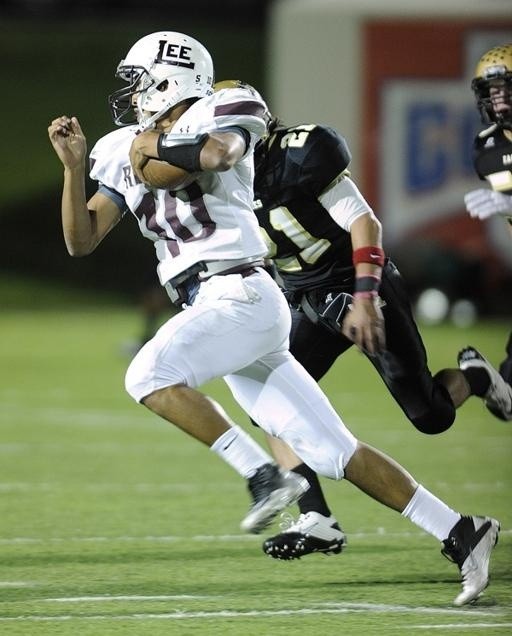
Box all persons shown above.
[465,45,512,386]
[212,80,512,561]
[47,30,501,607]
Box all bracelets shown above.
[354,276,380,297]
[352,246,384,267]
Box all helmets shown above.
[471,43,512,89]
[115,31,266,131]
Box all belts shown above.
[296,292,319,324]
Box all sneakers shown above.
[457,346,512,422]
[262,510,348,561]
[239,462,311,534]
[440,512,501,606]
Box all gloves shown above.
[342,298,387,358]
[463,188,512,221]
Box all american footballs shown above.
[142,157,203,191]
